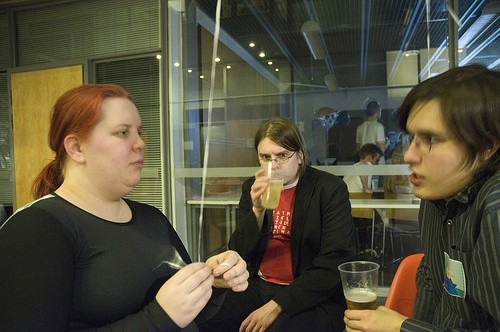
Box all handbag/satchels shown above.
[383,143,414,193]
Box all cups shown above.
[338,260,380,312]
[256,161,284,209]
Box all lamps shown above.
[301,19,327,61]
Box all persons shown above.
[343,63,500,332]
[0,83,215,332]
[329,109,357,163]
[342,143,384,226]
[356,101,387,166]
[199,115,360,332]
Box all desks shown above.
[186,196,421,249]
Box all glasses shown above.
[256,151,296,165]
[402,129,464,155]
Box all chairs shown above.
[348,192,424,287]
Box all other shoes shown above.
[358,249,377,260]
[375,247,384,258]
